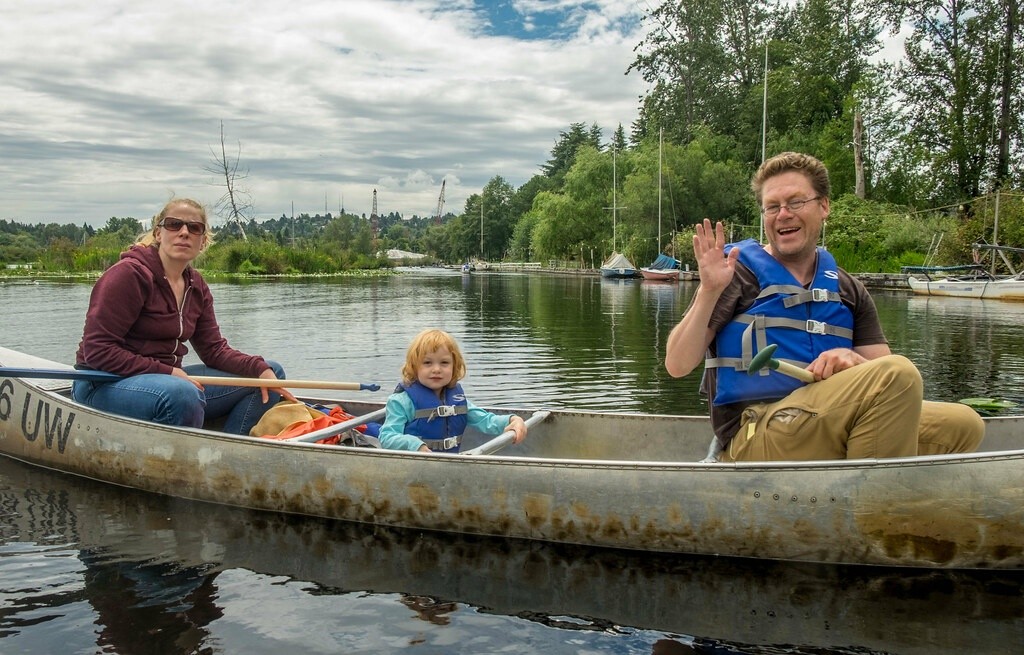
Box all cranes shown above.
[437,178,447,218]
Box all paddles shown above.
[747,342,822,384]
[0,367,382,393]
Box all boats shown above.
[908,190,1024,300]
[1,343,1024,574]
[0,448,1023,655]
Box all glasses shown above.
[761,197,822,218]
[156,217,205,235]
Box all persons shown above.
[379,329,527,452]
[665,152,986,463]
[70,198,298,436]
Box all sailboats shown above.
[640,127,696,281]
[599,131,640,279]
[472,203,493,273]
